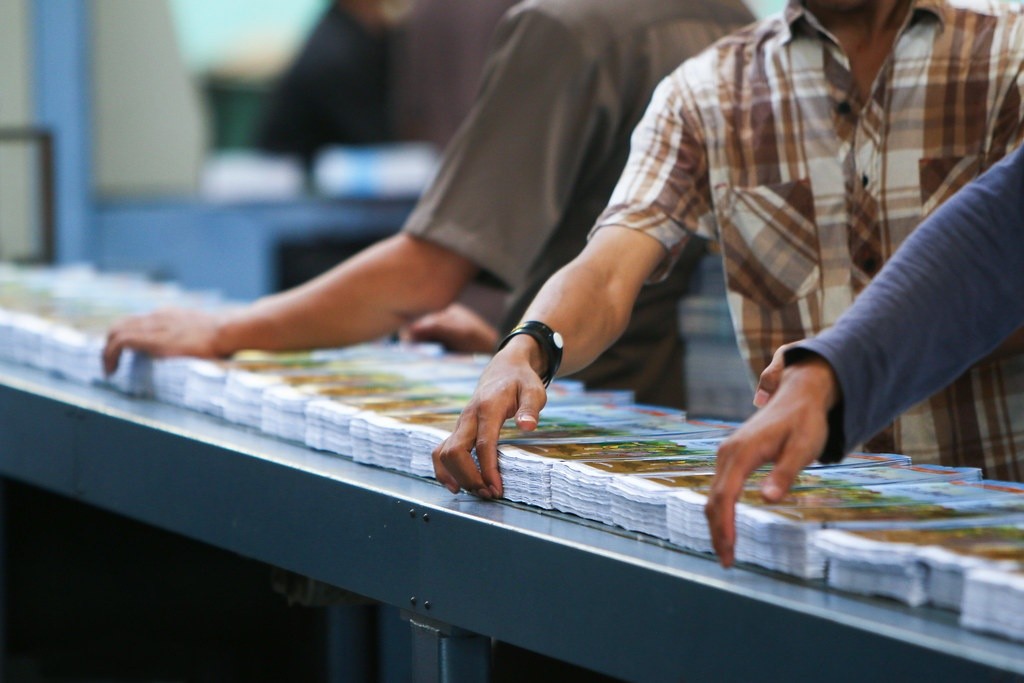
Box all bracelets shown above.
[491,321,563,390]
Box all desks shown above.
[85,193,421,311]
[0,356,1024,683]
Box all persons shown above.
[432,0,1024,499]
[103,1,757,411]
[705,140,1024,570]
